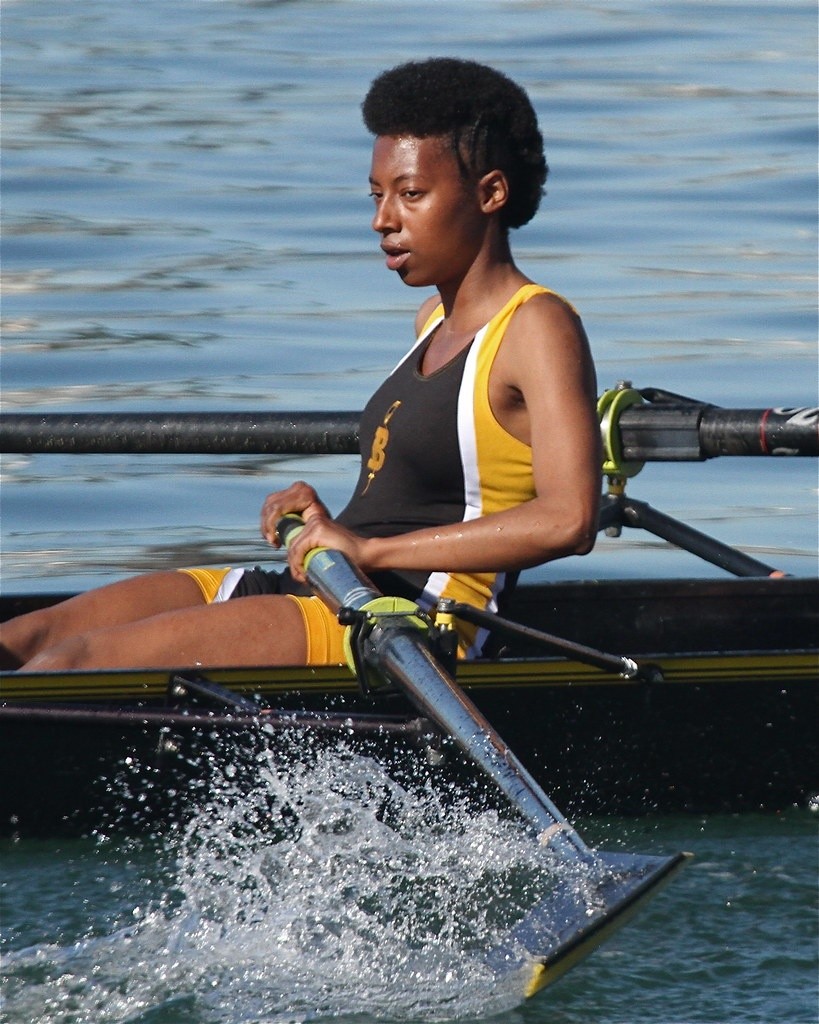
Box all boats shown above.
[1,408,819,830]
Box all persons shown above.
[0,57,600,672]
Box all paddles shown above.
[271,512,694,1004]
[0,403,819,462]
